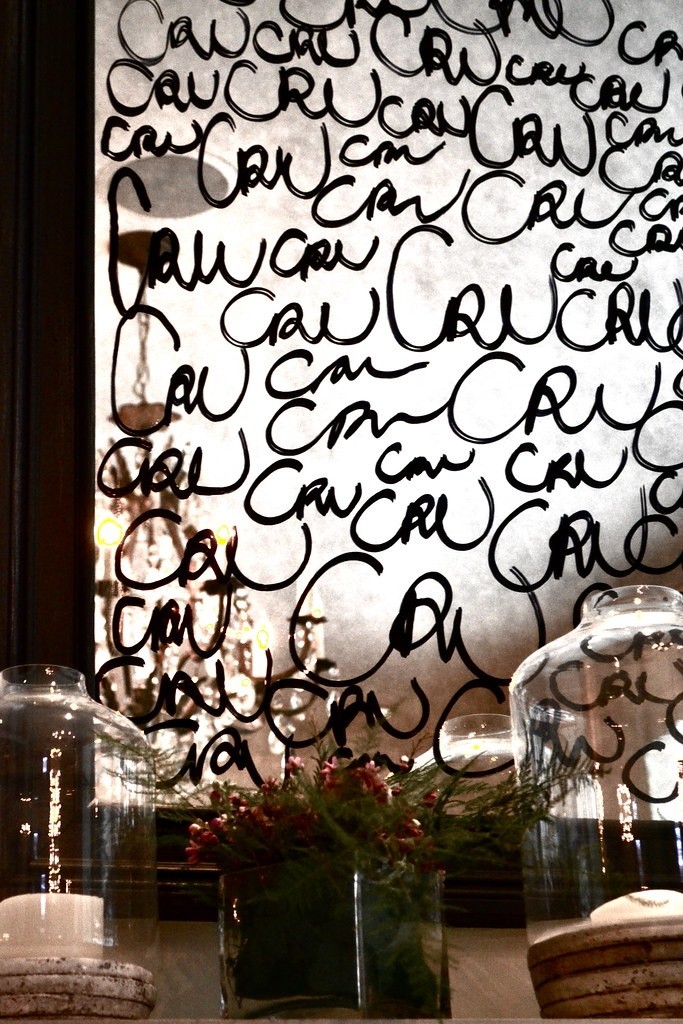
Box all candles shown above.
[589,890,683,923]
[0,893,104,961]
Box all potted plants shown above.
[84,692,614,1024]
